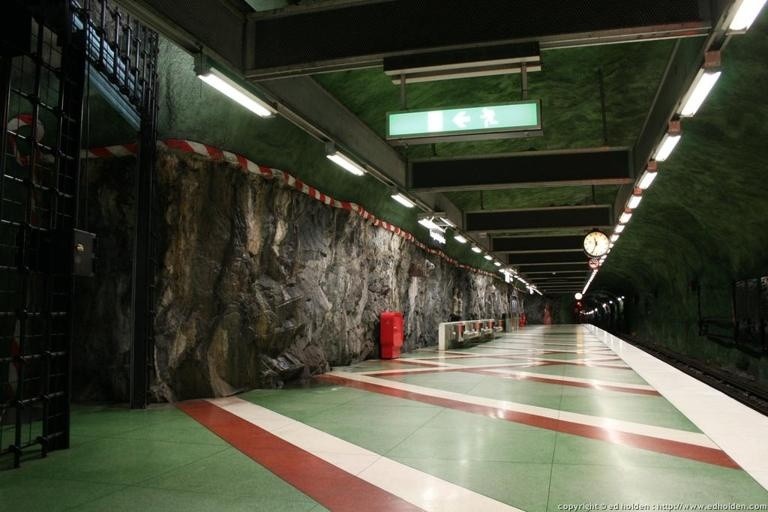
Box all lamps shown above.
[389,187,417,209]
[626,193,643,210]
[192,55,279,121]
[324,139,369,177]
[675,64,723,118]
[417,217,446,236]
[636,169,658,190]
[651,130,682,163]
[451,229,544,296]
[581,209,633,295]
[723,0,768,36]
